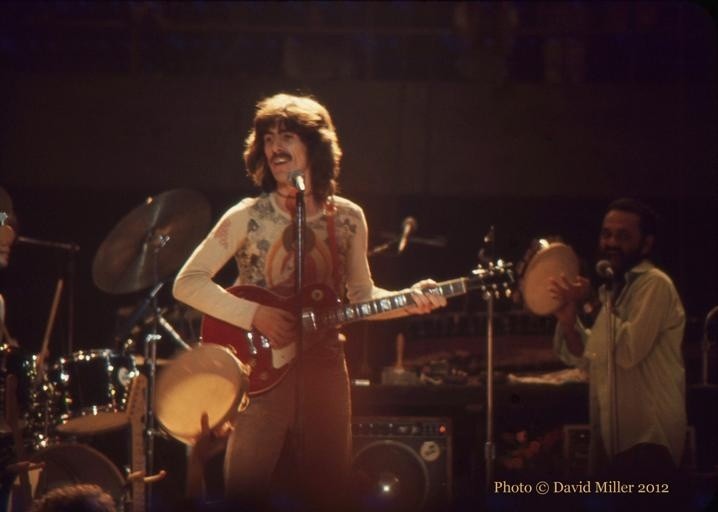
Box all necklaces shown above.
[274,188,314,199]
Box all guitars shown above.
[201,256,517,396]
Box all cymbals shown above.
[91,189,211,294]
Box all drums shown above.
[518,239,580,316]
[6,441,132,512]
[149,341,251,446]
[49,348,138,436]
[0,344,42,434]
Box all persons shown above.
[548,197,687,512]
[0,196,16,334]
[172,93,446,507]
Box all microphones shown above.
[595,259,615,280]
[286,168,308,194]
[396,215,417,254]
[478,224,496,263]
